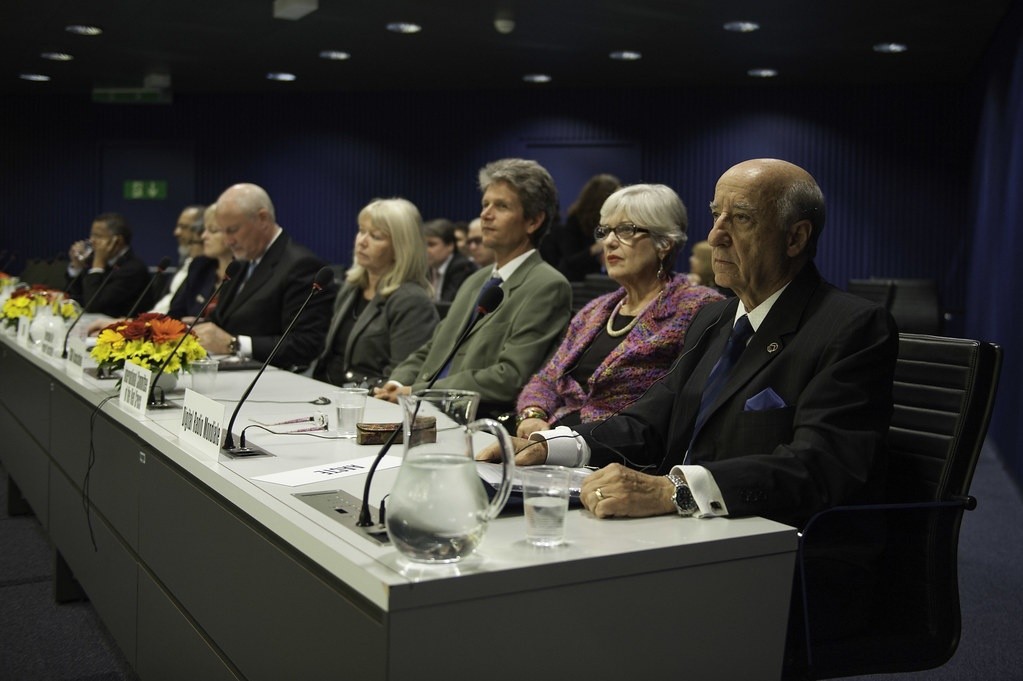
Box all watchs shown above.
[229,336,240,356]
[666,474,699,516]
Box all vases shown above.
[122,362,179,397]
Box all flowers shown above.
[1,283,76,328]
[91,312,211,379]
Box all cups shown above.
[188,359,219,394]
[334,387,369,435]
[523,464,572,546]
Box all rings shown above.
[596,489,604,499]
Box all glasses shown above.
[466,237,483,248]
[594,223,651,241]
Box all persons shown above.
[548,173,621,274]
[467,218,495,267]
[180,183,336,371]
[373,159,574,430]
[140,202,247,325]
[473,159,900,656]
[513,183,723,438]
[688,241,737,297]
[65,211,150,318]
[423,219,477,320]
[454,223,468,255]
[302,198,439,397]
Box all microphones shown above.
[126,257,171,322]
[64,253,95,293]
[224,266,336,450]
[358,285,504,527]
[148,261,243,404]
[62,259,126,358]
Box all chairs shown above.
[433,262,1004,681]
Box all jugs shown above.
[385,389,515,564]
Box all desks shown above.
[0,315,800,681]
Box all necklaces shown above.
[353,305,357,320]
[606,295,659,337]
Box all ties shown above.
[679,313,754,465]
[233,262,257,301]
[432,268,441,300]
[434,276,503,380]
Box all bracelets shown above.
[516,408,549,425]
